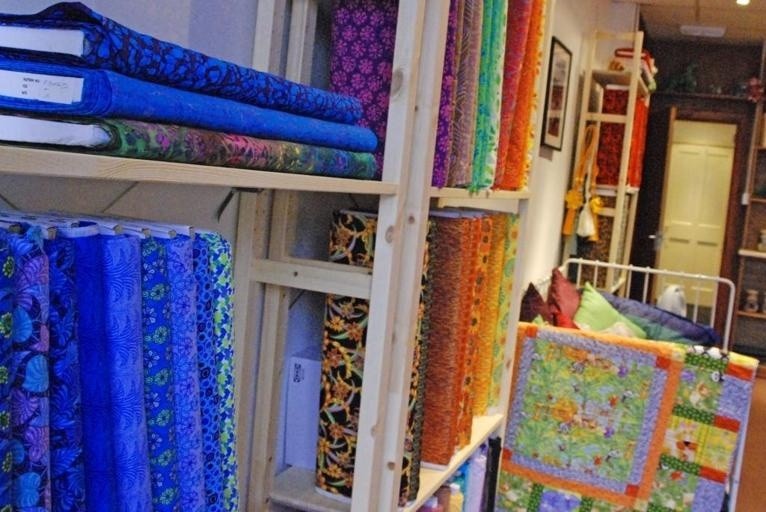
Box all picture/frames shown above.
[541,36,572,151]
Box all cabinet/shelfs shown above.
[573,27,652,292]
[735,149,766,319]
[0,0,553,511]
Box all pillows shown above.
[547,269,582,319]
[572,283,647,339]
[551,305,579,328]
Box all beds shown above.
[494,258,760,512]
